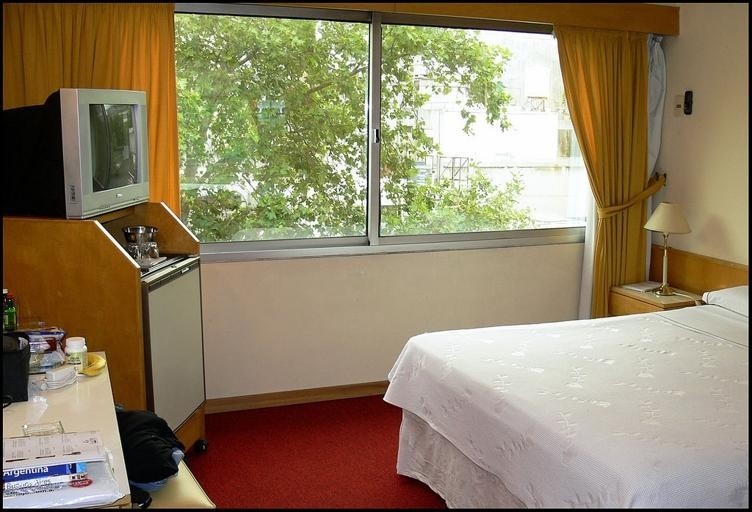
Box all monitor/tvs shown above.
[3,88,149,219]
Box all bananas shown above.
[84,350,106,376]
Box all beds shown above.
[378,273,750,510]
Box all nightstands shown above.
[605,278,702,319]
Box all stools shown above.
[139,449,220,510]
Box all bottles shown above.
[3,287,18,332]
[64,336,89,373]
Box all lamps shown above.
[642,201,692,296]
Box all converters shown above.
[45,365,74,381]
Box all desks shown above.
[0,341,136,510]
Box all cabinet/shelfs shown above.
[2,199,217,461]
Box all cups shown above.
[42,336,57,354]
[127,242,161,269]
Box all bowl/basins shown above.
[122,224,160,243]
